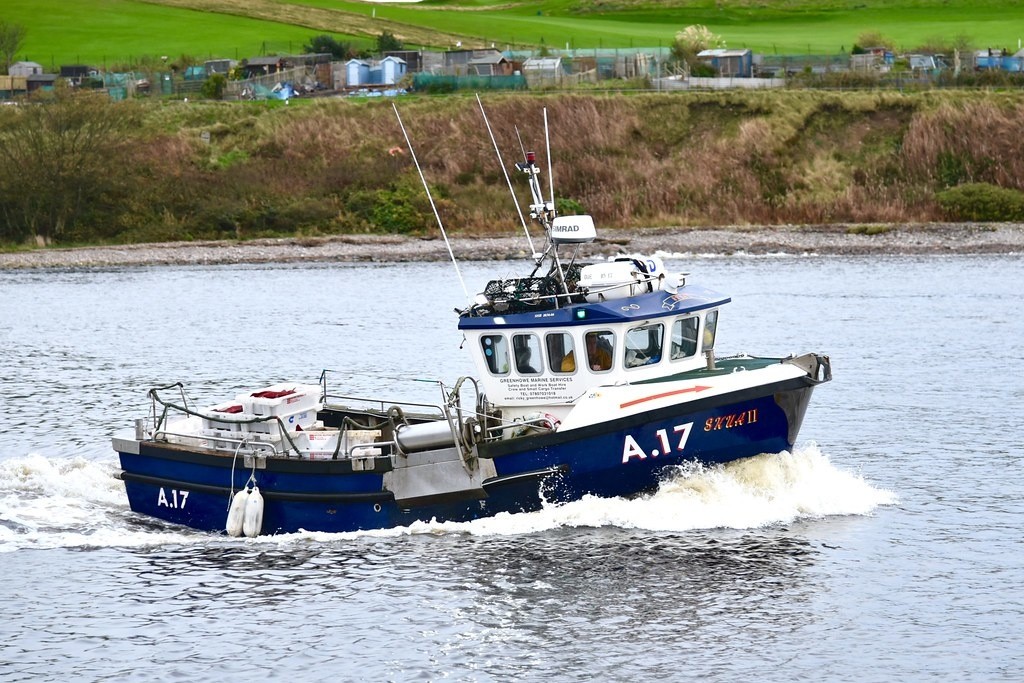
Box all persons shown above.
[500,347,537,373]
[561,335,611,372]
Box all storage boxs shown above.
[202,383,381,458]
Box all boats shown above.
[102,90,835,538]
